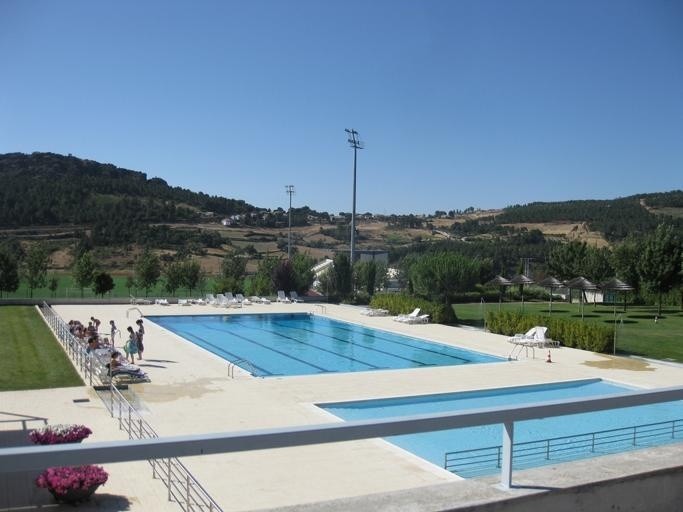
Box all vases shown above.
[46,486,99,504]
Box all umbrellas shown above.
[485,273,634,321]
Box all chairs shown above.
[154,298,169,305]
[177,292,270,308]
[103,364,148,383]
[128,295,152,305]
[276,291,304,303]
[392,308,429,325]
[508,325,560,349]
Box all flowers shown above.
[33,462,109,496]
[25,423,91,445]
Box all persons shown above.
[66,316,144,373]
